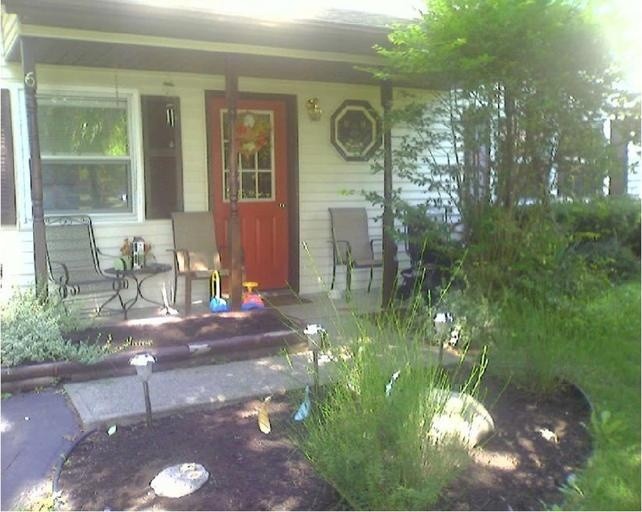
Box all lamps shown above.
[306,96,323,121]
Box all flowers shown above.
[119,236,150,256]
[234,113,266,160]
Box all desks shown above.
[103,262,172,315]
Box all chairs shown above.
[329,207,384,292]
[166,211,245,317]
[43,215,129,321]
